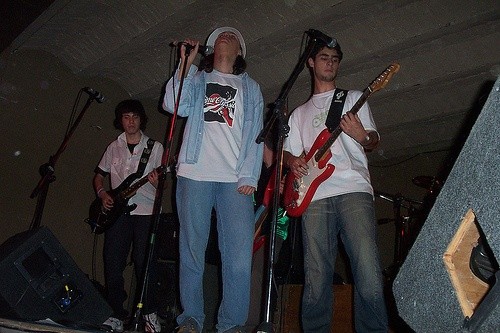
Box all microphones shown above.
[86,87,106,103]
[173,40,214,55]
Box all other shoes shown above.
[222,325,250,333]
[178,316,200,333]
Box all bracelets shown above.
[96,184,106,198]
[360,134,374,146]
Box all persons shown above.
[93,98,171,333]
[283,36,391,333]
[163,27,265,333]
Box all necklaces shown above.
[311,97,332,109]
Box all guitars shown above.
[89,154,178,234]
[252,174,287,251]
[284,63,401,217]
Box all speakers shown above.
[147,259,222,333]
[394,75,500,333]
[0,223,115,328]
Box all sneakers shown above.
[135,313,161,333]
[101,318,124,333]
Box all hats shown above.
[206,26,246,59]
[308,30,343,64]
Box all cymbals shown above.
[411,177,445,192]
